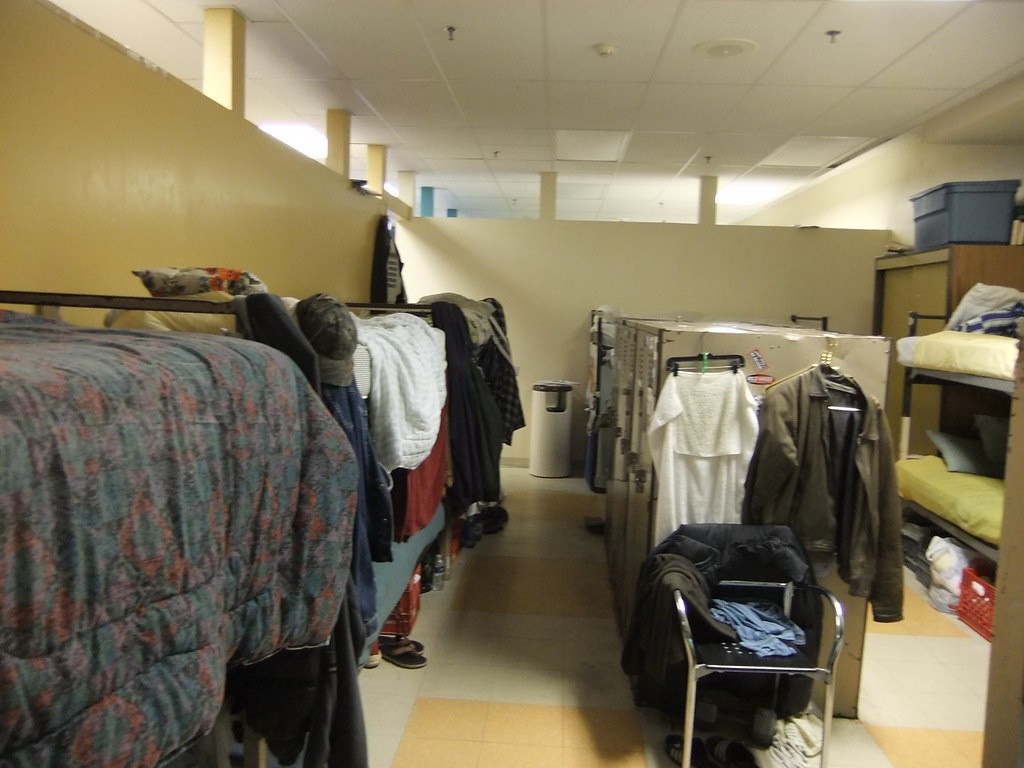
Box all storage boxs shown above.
[381,561,423,637]
[955,569,999,641]
[909,177,1021,248]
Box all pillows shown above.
[129,267,268,295]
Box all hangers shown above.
[667,333,873,413]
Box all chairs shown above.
[669,521,846,767]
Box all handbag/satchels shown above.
[925,535,989,613]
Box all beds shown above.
[899,307,1023,612]
[1,288,528,767]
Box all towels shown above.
[644,370,762,549]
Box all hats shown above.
[296,293,358,386]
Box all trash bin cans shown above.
[529,382,573,477]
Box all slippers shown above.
[706,735,760,768]
[665,734,722,768]
[379,634,424,655]
[381,644,428,668]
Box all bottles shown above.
[431,554,445,590]
